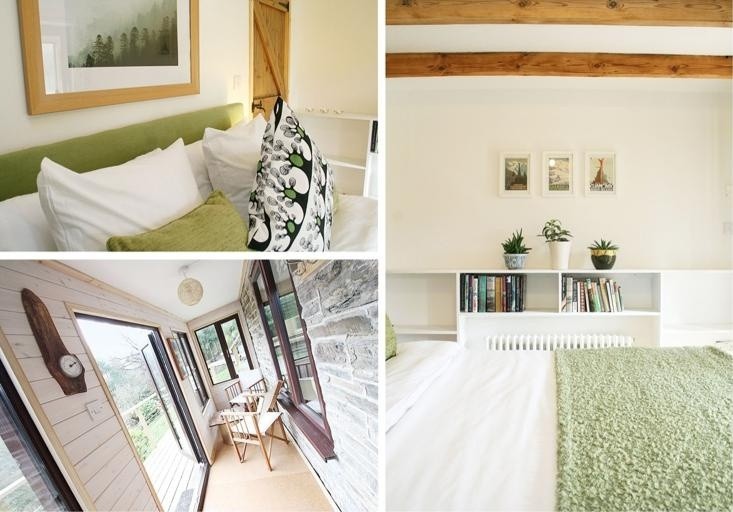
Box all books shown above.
[562,276,625,312]
[460,273,527,312]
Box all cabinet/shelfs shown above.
[295,111,375,197]
[456,270,662,348]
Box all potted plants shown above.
[501,227,533,269]
[536,218,574,270]
[587,237,620,269]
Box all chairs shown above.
[220,378,289,471]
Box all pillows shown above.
[386,315,396,358]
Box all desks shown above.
[209,408,248,445]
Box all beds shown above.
[385,341,732,511]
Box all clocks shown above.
[59,354,82,379]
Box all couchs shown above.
[1,102,376,253]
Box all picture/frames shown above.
[17,1,201,116]
[541,149,575,197]
[584,151,618,197]
[498,150,532,197]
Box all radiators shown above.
[486,335,634,350]
